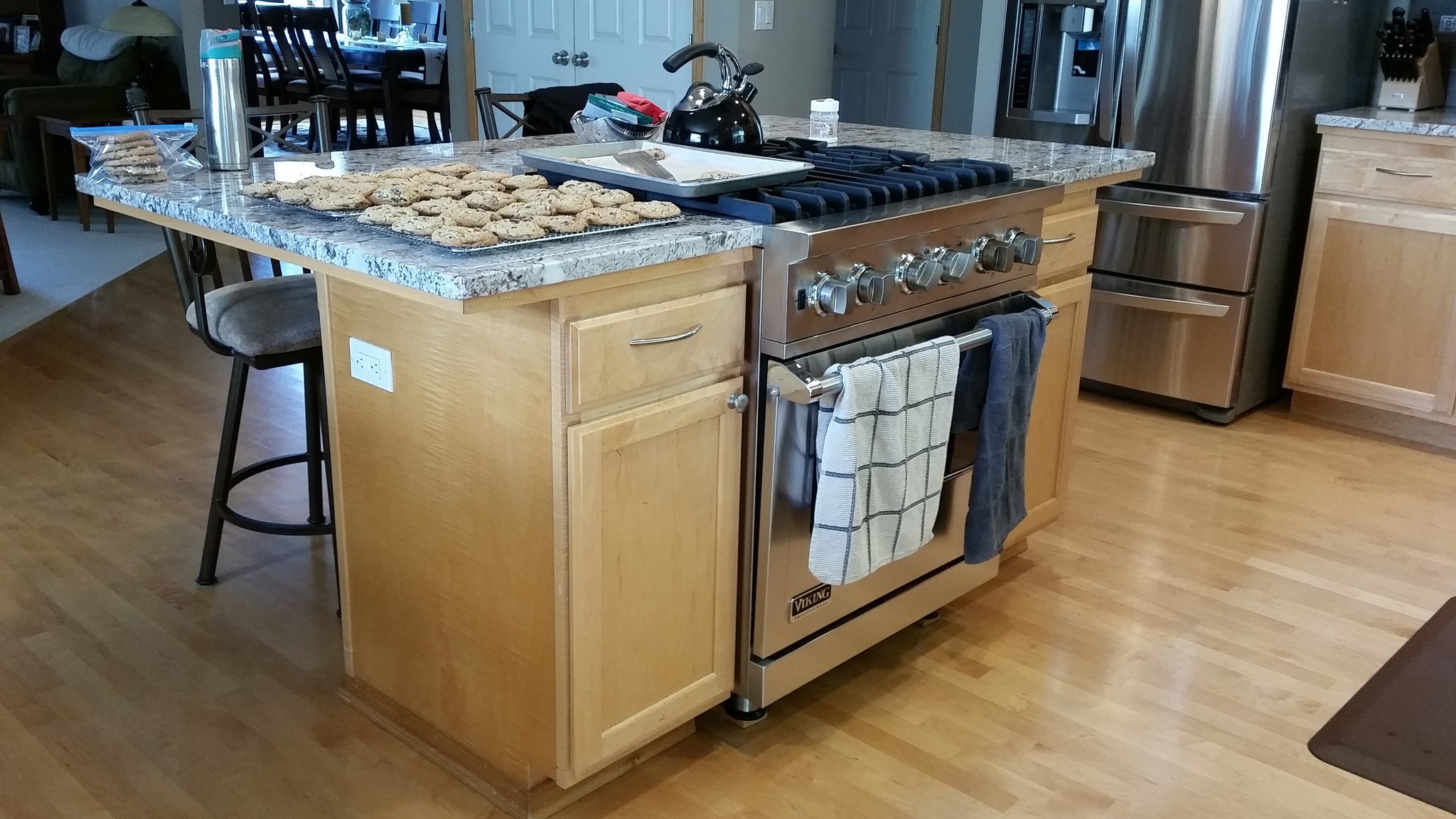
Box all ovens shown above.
[727,272,1058,725]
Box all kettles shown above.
[662,40,765,153]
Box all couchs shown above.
[0,25,177,215]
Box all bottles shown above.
[809,98,839,143]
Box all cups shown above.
[418,33,427,44]
[351,30,361,41]
[377,31,386,42]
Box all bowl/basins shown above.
[570,110,662,145]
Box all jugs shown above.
[341,0,373,41]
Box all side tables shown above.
[37,114,135,234]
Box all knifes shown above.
[1377,7,1434,82]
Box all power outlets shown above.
[349,337,393,393]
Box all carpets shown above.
[1,196,189,344]
[1307,595,1456,818]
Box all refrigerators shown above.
[992,0,1391,429]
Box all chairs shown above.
[125,80,342,617]
[366,0,450,145]
[236,0,388,152]
[475,81,623,140]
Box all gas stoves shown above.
[512,133,1065,359]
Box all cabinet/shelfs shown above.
[999,187,1103,568]
[1281,125,1456,451]
[311,250,751,819]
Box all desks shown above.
[253,31,450,151]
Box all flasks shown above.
[199,28,251,171]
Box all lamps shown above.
[97,0,184,114]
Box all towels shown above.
[808,336,962,585]
[963,307,1049,564]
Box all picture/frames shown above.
[0,14,41,53]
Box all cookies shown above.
[93,128,169,185]
[240,148,739,247]
